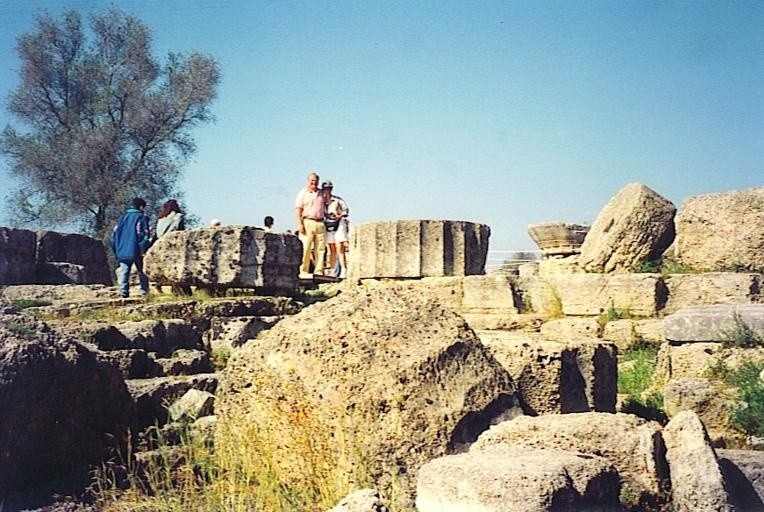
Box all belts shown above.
[305,217,324,222]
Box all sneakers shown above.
[315,271,324,276]
[339,269,348,278]
[299,273,313,280]
[326,269,337,277]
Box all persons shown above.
[110,197,156,298]
[263,215,274,230]
[286,229,350,277]
[153,199,185,294]
[320,180,351,279]
[293,172,327,277]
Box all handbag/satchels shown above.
[325,220,339,232]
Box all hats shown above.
[322,180,333,188]
